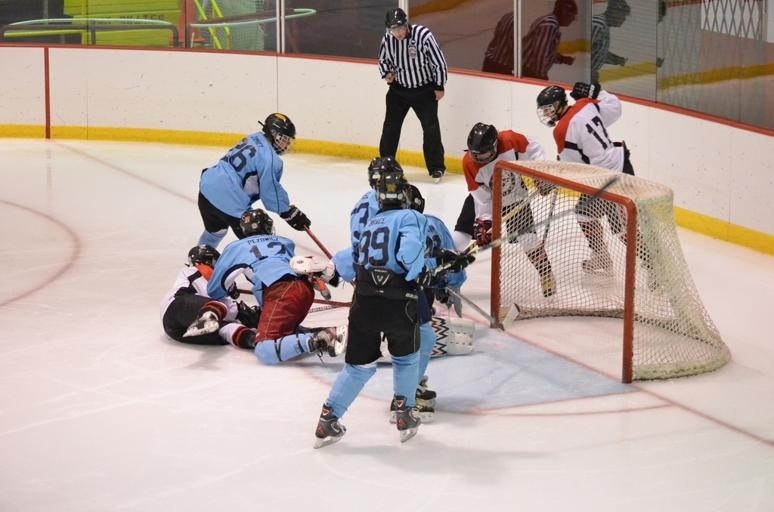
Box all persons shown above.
[591,0,631,85]
[206,210,349,366]
[522,1,578,80]
[482,11,514,74]
[455,123,567,298]
[197,111,311,247]
[311,172,470,449]
[160,243,331,351]
[290,184,467,425]
[536,81,662,292]
[378,7,448,178]
[350,156,404,270]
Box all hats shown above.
[187,243,220,269]
[535,84,567,129]
[367,156,402,189]
[466,122,499,166]
[385,7,408,28]
[257,112,296,153]
[238,210,274,236]
[403,185,424,213]
[375,172,404,205]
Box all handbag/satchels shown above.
[352,263,413,300]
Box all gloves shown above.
[570,81,601,100]
[437,248,476,274]
[416,264,446,290]
[473,218,494,247]
[289,254,339,288]
[237,299,261,327]
[277,203,312,233]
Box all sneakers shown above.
[389,395,422,431]
[306,326,347,367]
[581,255,614,270]
[186,311,220,334]
[414,378,437,401]
[538,272,557,298]
[315,402,347,439]
[429,166,446,177]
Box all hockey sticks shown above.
[444,286,519,332]
